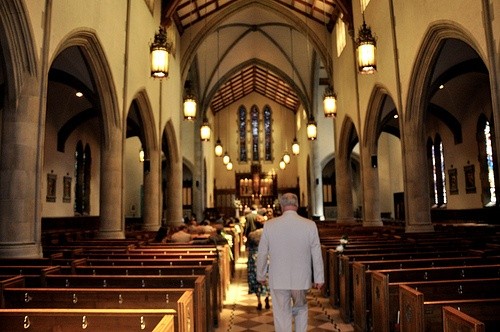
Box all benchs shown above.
[314,221,500,332]
[0,223,238,332]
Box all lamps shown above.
[149,0,377,169]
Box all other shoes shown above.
[265,297,270,308]
[256,304,262,310]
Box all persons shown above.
[255,193,325,332]
[153,193,325,332]
